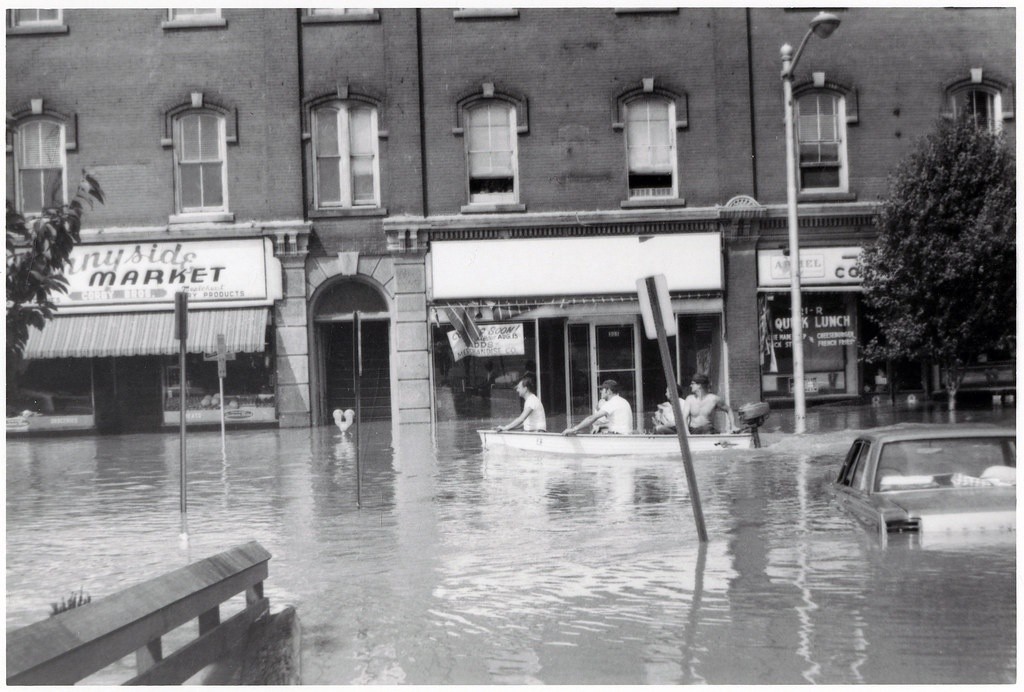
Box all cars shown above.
[819,420,1017,548]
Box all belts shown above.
[529,430,545,432]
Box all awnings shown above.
[25,307,282,357]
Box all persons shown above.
[560,373,740,436]
[495,376,547,434]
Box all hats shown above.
[692,373,709,384]
[597,380,617,393]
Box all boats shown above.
[472,426,760,452]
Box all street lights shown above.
[781,12,842,437]
